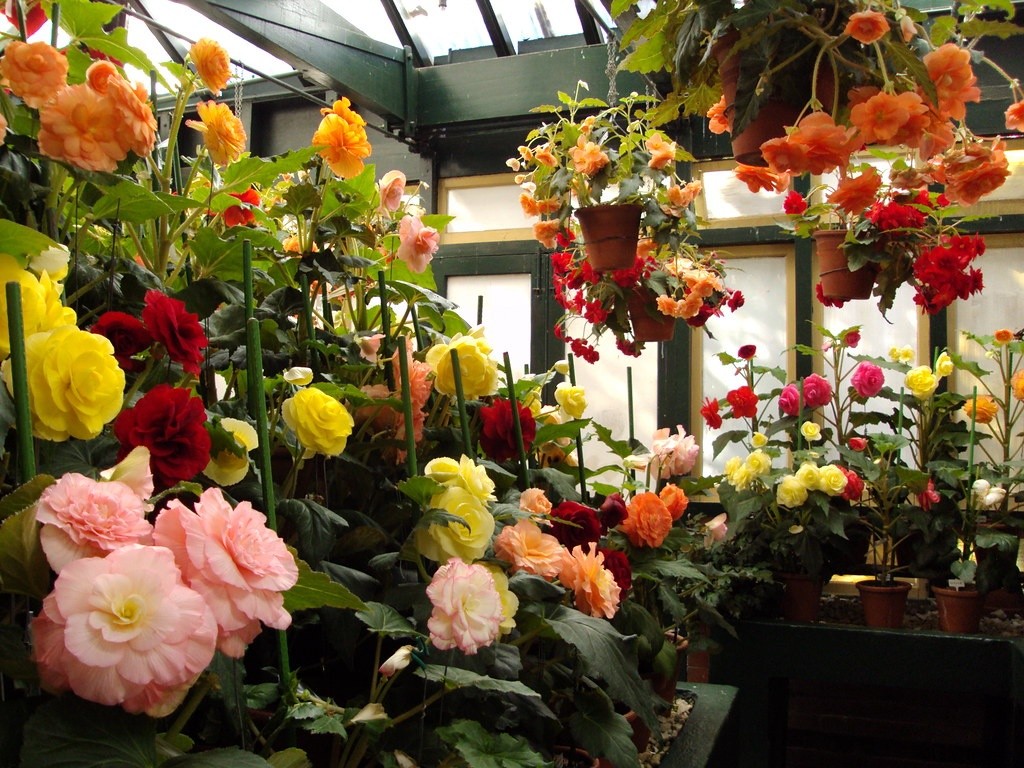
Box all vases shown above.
[575,205,641,271]
[813,228,887,301]
[855,579,912,630]
[711,29,834,168]
[776,573,822,620]
[615,703,651,754]
[627,286,675,342]
[644,632,688,704]
[931,586,985,634]
[550,745,602,767]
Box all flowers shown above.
[0,1,1024,768]
